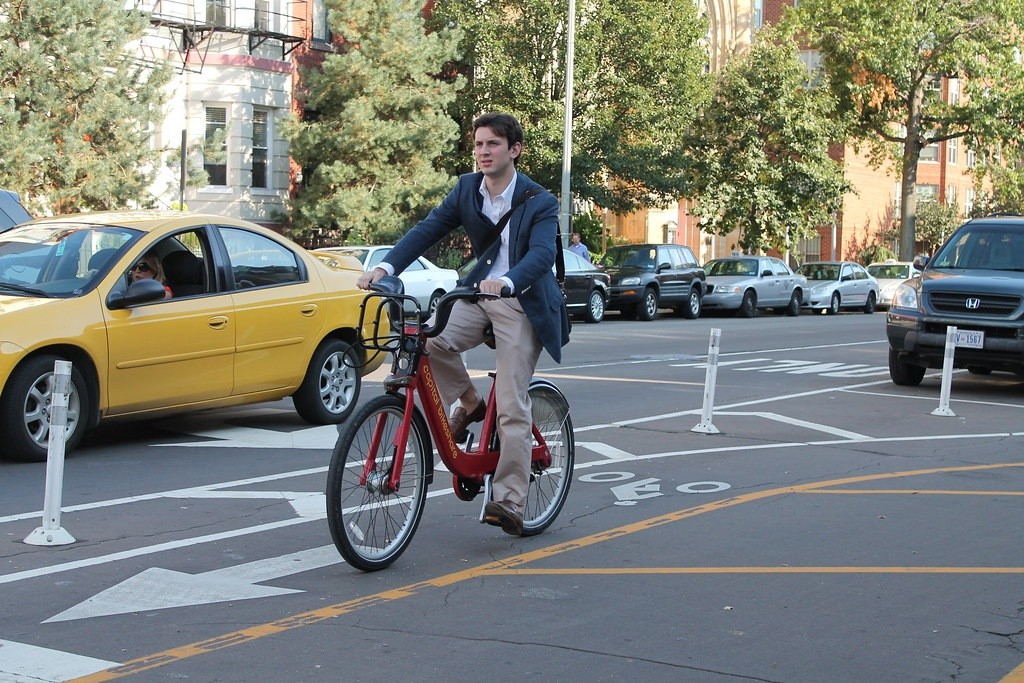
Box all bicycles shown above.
[323,274,576,573]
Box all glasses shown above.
[129,263,150,271]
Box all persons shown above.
[566,232,592,264]
[130,252,174,299]
[357,115,572,534]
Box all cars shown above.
[457,246,612,324]
[315,244,460,324]
[0,204,392,460]
[703,250,811,316]
[795,261,881,315]
[867,258,922,311]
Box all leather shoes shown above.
[484,501,524,536]
[447,396,488,440]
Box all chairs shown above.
[162,250,203,298]
[982,242,1016,269]
[88,249,119,271]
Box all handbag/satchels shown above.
[474,185,573,335]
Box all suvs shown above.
[886,212,1024,386]
[596,243,706,322]
